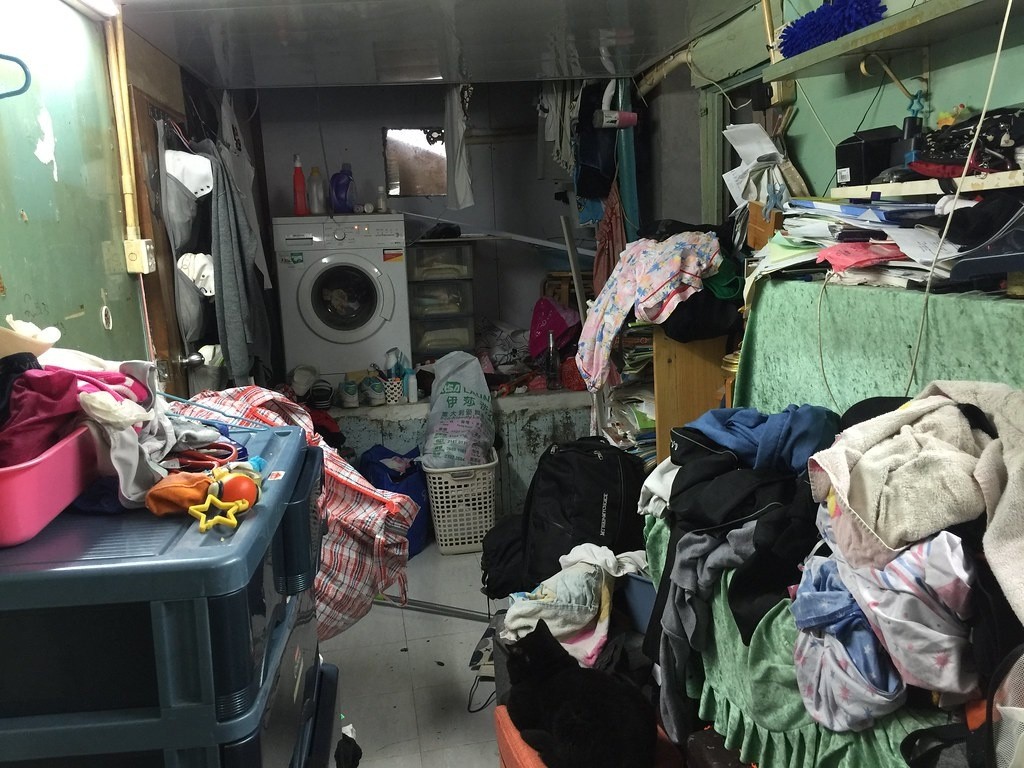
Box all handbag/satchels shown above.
[164,385,421,642]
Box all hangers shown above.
[224,77,232,107]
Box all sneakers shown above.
[358,377,386,406]
[332,381,359,408]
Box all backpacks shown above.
[520,435,646,589]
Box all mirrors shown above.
[385,127,447,196]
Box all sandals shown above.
[310,379,333,408]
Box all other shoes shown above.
[296,389,310,408]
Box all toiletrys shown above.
[403,368,417,403]
[385,347,400,378]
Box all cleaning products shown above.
[292,154,310,216]
[328,163,356,213]
[306,166,327,217]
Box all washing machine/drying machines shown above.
[272,213,412,391]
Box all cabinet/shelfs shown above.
[598,314,733,479]
[407,243,476,398]
[760,0,1024,199]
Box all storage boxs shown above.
[0,424,92,546]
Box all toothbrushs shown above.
[367,362,388,382]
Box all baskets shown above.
[422,446,500,555]
